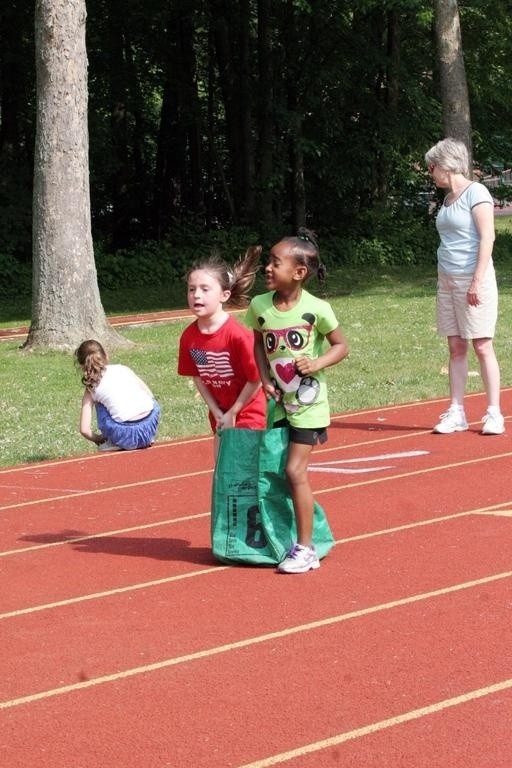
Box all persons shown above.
[239,225,350,575]
[423,137,506,435]
[176,244,267,464]
[74,340,161,452]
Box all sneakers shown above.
[434,408,469,433]
[481,411,505,434]
[278,543,320,573]
[98,441,121,450]
